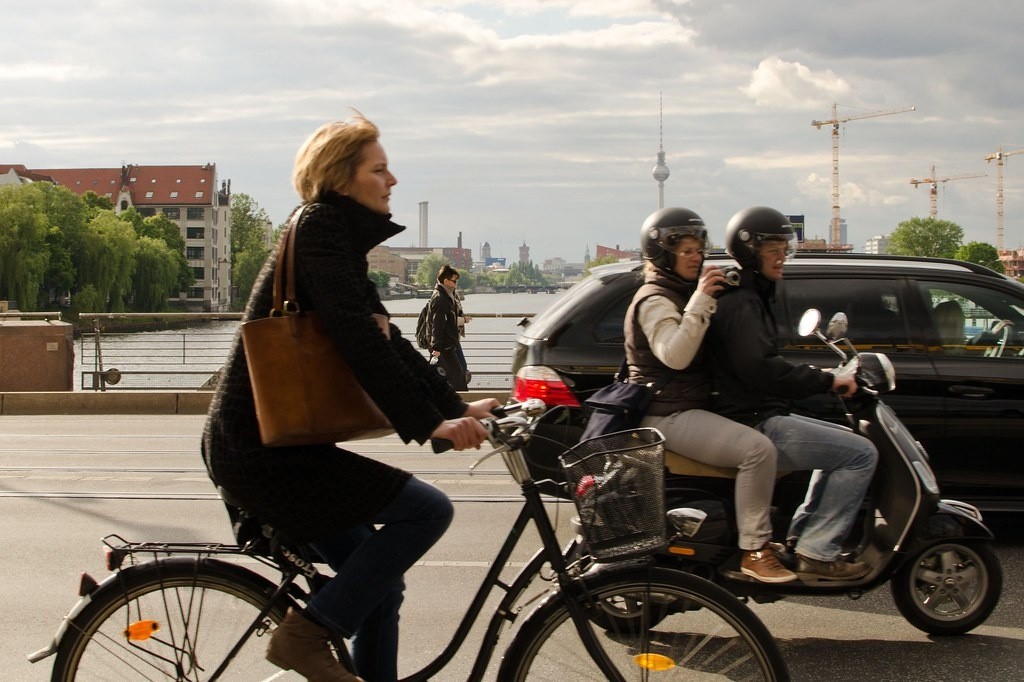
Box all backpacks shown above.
[416,291,439,349]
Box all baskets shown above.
[557,427,667,563]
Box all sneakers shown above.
[739,544,797,584]
[798,556,870,583]
[266,606,365,682]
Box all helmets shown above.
[641,208,708,270]
[724,207,798,273]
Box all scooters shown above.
[550,309,1004,635]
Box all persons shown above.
[691,202,880,581]
[200,117,503,682]
[902,286,1014,345]
[623,205,798,583]
[423,264,467,391]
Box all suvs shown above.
[511,247,1024,516]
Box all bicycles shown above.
[26,397,791,682]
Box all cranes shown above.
[982,142,1023,254]
[812,102,917,243]
[909,165,989,218]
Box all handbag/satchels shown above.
[579,381,652,447]
[241,202,397,446]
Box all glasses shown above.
[449,279,457,284]
